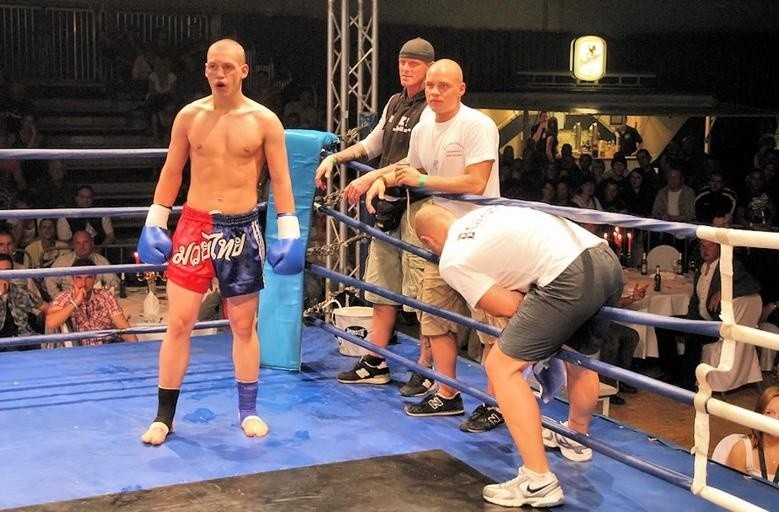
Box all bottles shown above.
[144,292,159,321]
[120,272,128,298]
[593,140,606,157]
[640,253,696,292]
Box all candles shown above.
[134,252,140,264]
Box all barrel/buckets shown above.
[328,301,373,360]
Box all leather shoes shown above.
[618,380,638,394]
[610,394,626,405]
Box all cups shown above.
[395,162,409,176]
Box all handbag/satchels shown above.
[375,198,403,231]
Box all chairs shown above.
[60,320,81,348]
[647,245,683,274]
[696,294,763,399]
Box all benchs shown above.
[526,374,619,419]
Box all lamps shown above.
[569,33,607,82]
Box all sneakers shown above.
[402,392,464,417]
[459,401,506,435]
[337,354,394,385]
[539,415,593,462]
[399,364,435,398]
[481,465,566,508]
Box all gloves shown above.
[265,211,306,277]
[136,202,174,266]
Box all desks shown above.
[611,269,694,359]
[111,286,219,341]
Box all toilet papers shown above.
[703,115,713,156]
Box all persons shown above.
[255,68,320,128]
[198,288,231,333]
[132,43,207,143]
[1,82,136,350]
[306,36,639,507]
[137,39,305,446]
[604,148,777,397]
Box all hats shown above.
[398,35,436,61]
